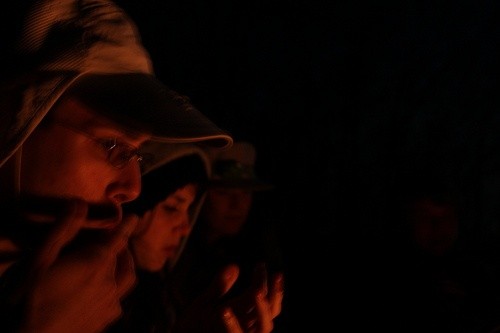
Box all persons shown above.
[195,136,270,239]
[404,184,462,295]
[0,1,234,333]
[100,131,287,332]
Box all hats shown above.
[70,73,233,153]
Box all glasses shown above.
[209,158,257,188]
